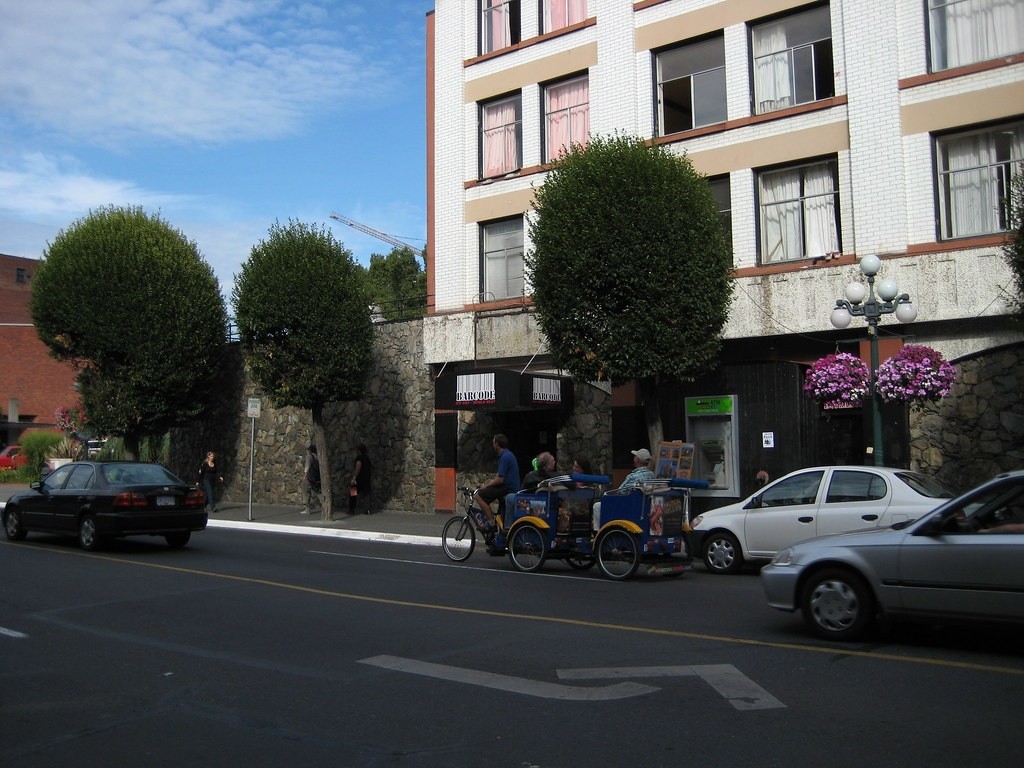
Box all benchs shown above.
[514,486,685,540]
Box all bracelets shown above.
[352,478,357,480]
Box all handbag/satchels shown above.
[305,453,320,485]
[350,487,357,496]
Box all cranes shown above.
[329,211,427,259]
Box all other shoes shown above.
[490,549,505,556]
[345,511,354,515]
[212,508,218,513]
[476,521,498,532]
[301,508,310,515]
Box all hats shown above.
[631,449,651,462]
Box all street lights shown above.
[829,253,919,467]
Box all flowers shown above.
[803,352,872,402]
[874,345,957,402]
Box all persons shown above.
[195,451,224,514]
[978,523,1024,536]
[618,448,655,495]
[474,434,520,531]
[522,451,563,489]
[573,454,593,486]
[350,444,371,513]
[300,443,322,513]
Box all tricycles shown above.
[441,471,716,583]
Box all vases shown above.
[824,398,862,409]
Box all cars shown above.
[0,446,30,469]
[3,461,208,553]
[85,438,115,456]
[686,467,986,575]
[759,470,1024,647]
